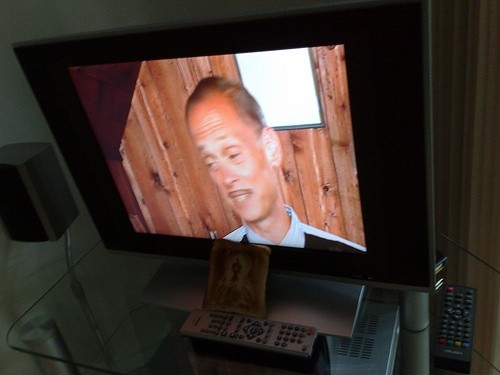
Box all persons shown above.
[183,76,368,254]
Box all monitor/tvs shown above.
[10,1,436,293]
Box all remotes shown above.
[433,281,477,368]
[179,308,319,360]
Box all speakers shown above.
[1,143,80,243]
